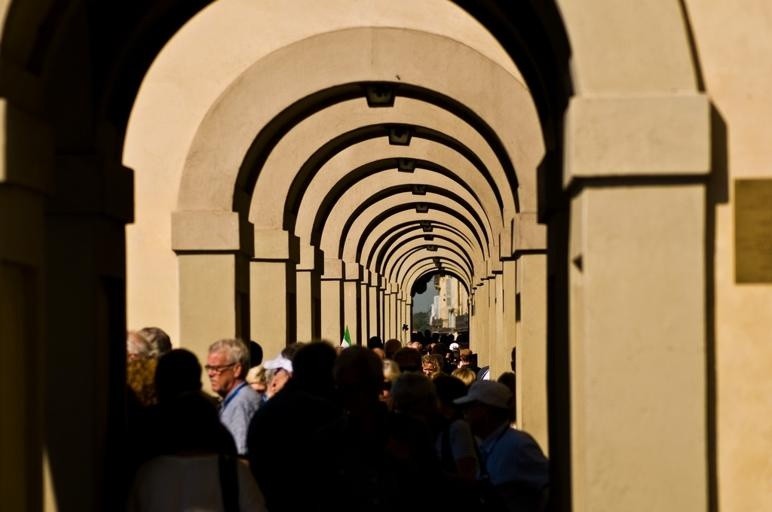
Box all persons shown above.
[130,327,550,511]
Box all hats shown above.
[452,380,511,409]
[262,356,293,373]
[449,343,459,351]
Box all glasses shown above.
[205,362,236,373]
